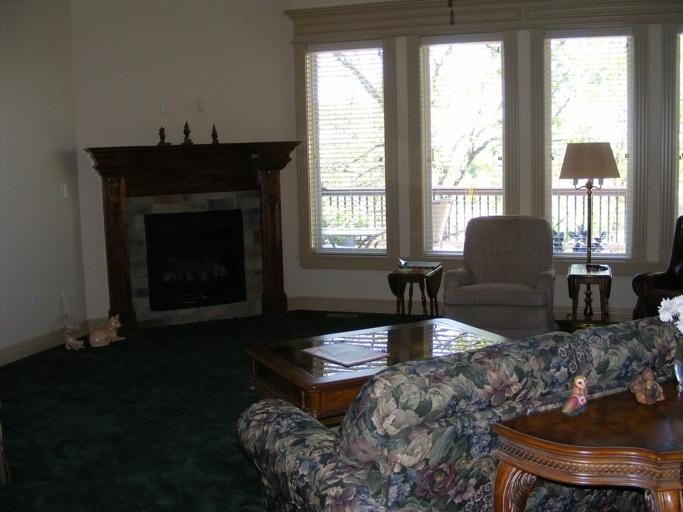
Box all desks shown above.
[565,262,612,328]
[485,374,682,512]
[385,259,442,322]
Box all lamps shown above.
[558,141,620,324]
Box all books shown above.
[301,343,391,367]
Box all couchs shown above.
[235,314,682,512]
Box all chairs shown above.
[631,215,681,319]
[438,215,557,345]
